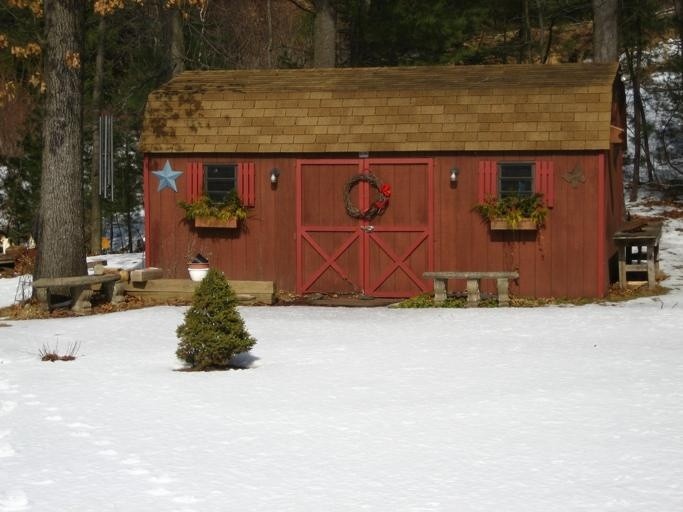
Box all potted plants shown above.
[174,264,258,371]
[175,191,256,230]
[185,252,210,269]
[468,190,553,232]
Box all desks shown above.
[611,218,665,290]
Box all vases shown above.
[188,269,209,282]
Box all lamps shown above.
[269,167,280,188]
[448,166,458,185]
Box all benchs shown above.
[421,270,520,308]
